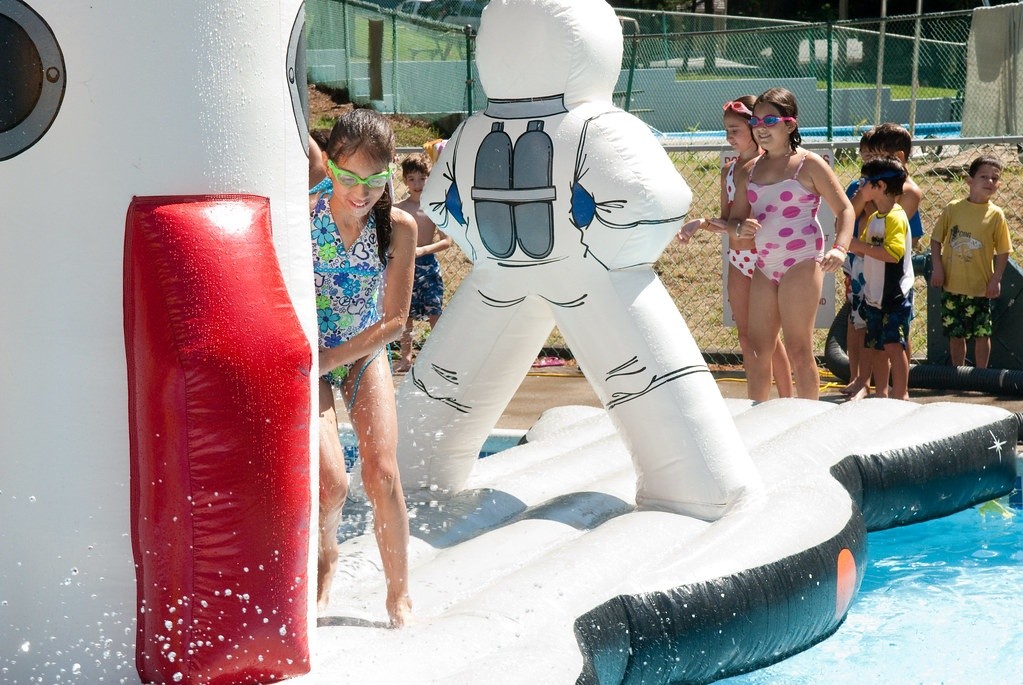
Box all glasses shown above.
[858,173,898,188]
[722,101,753,116]
[327,158,391,191]
[751,114,795,128]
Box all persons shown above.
[675,94,796,399]
[875,121,925,362]
[840,123,886,400]
[301,107,420,638]
[930,153,1013,367]
[726,90,855,400]
[392,151,454,373]
[839,156,917,400]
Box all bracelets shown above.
[734,221,740,240]
